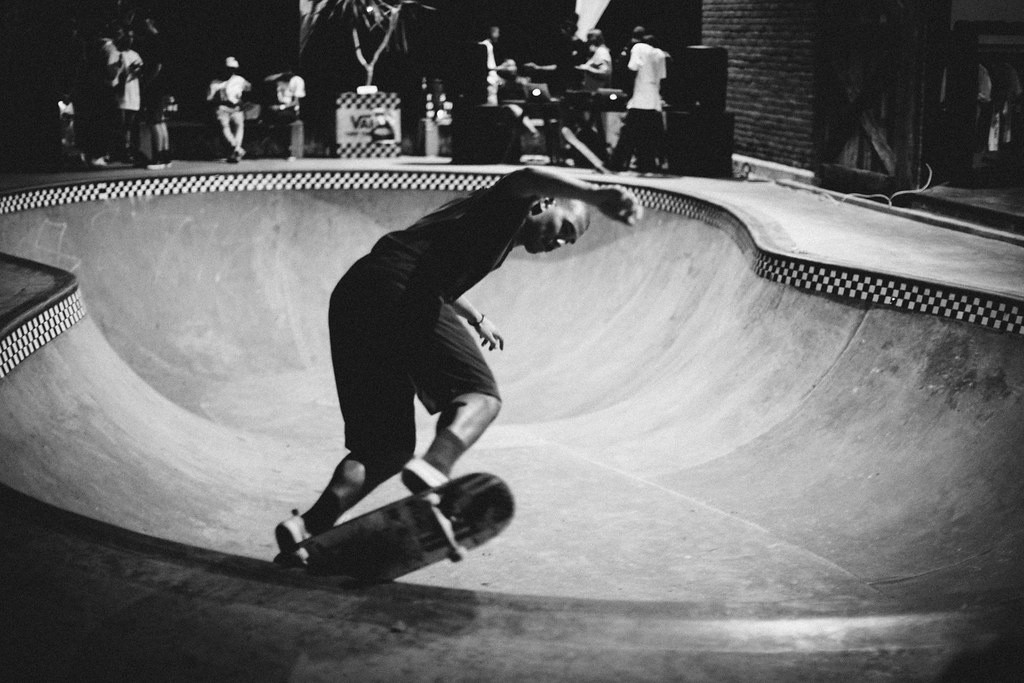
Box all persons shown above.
[603,24,675,173]
[42,20,614,163]
[273,166,644,567]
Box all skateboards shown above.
[273,473,516,581]
[560,127,612,175]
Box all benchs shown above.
[136,119,305,166]
[418,115,453,156]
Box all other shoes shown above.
[147,150,172,170]
[227,149,246,162]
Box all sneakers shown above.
[402,458,449,495]
[276,509,313,552]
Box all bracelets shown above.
[467,312,486,325]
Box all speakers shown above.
[450,104,540,166]
[664,46,736,179]
